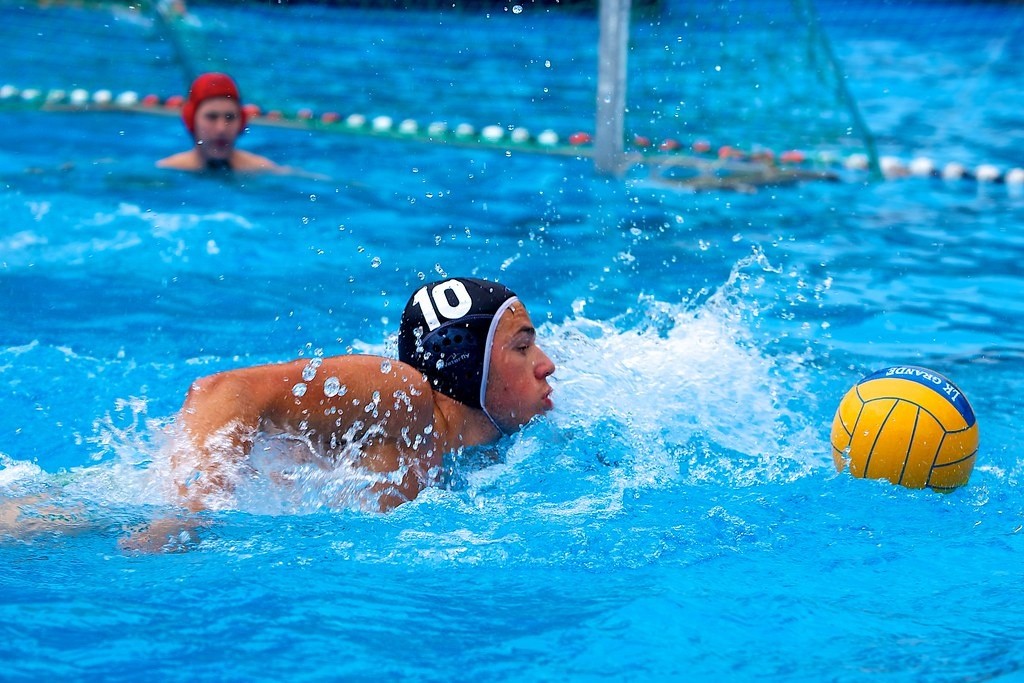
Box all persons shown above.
[153,73,278,172]
[153,278,555,510]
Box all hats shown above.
[182,74,245,134]
[398,277,518,437]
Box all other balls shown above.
[829,366,980,494]
